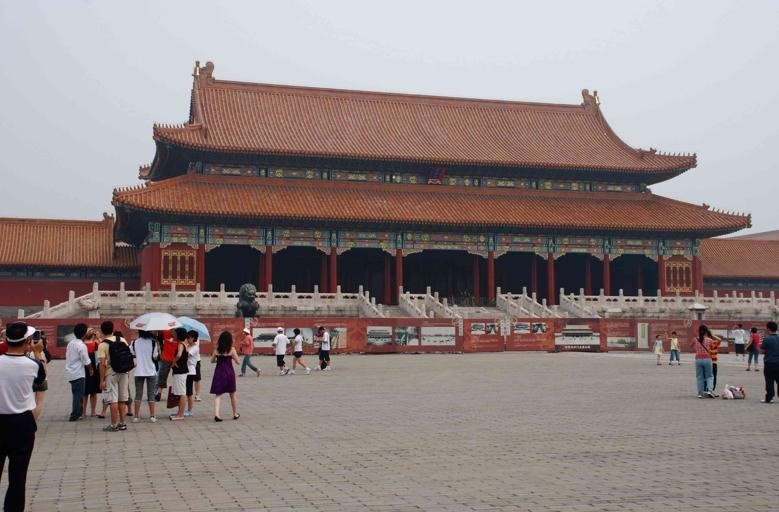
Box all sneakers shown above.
[102,415,157,432]
[279,365,331,376]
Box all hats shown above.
[277,326,284,332]
[6,325,37,342]
[243,328,250,334]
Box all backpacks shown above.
[103,335,134,373]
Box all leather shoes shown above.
[215,416,223,422]
[234,413,240,419]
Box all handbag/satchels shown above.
[95,342,101,370]
[167,386,180,408]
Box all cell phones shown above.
[32,330,41,342]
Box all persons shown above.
[688,323,715,399]
[272,328,312,376]
[65,321,202,431]
[0,321,47,511]
[239,328,262,377]
[652,334,665,366]
[210,331,240,422]
[731,323,746,362]
[760,320,779,403]
[315,326,332,371]
[743,327,761,372]
[665,330,681,365]
[702,329,721,397]
